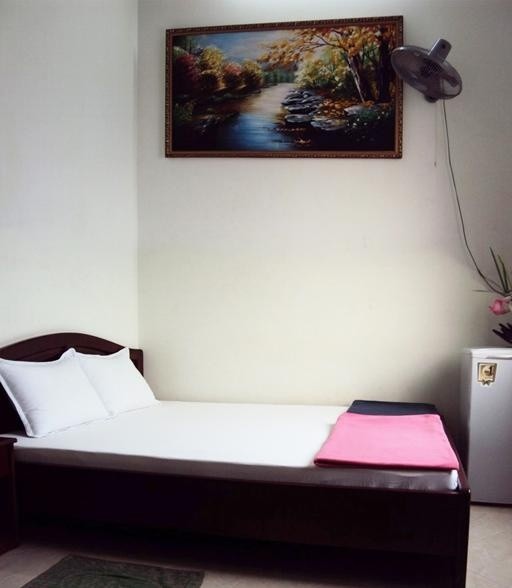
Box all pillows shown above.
[1,347,157,438]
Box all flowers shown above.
[473,242,512,347]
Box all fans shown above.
[389,37,464,103]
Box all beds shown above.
[0,332,470,588]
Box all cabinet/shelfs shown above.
[459,347,512,506]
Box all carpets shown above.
[21,554,205,588]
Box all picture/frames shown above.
[165,15,403,159]
